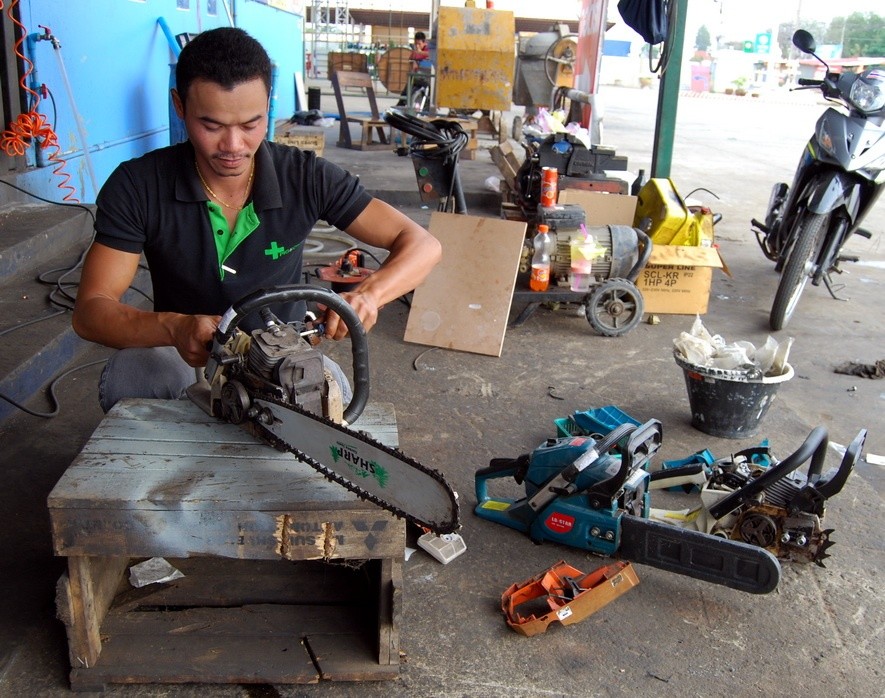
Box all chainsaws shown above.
[187,284,464,538]
[471,418,782,595]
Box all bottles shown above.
[530,225,552,292]
[631,170,646,195]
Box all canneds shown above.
[541,167,557,207]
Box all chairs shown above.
[333,70,396,152]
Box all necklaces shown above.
[194,156,255,210]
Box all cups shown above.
[570,241,592,292]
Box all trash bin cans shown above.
[674,343,795,440]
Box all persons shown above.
[396,31,433,107]
[72,28,442,415]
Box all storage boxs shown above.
[45,396,405,693]
[635,177,730,313]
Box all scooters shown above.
[749,28,885,332]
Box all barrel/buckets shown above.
[673,347,794,439]
